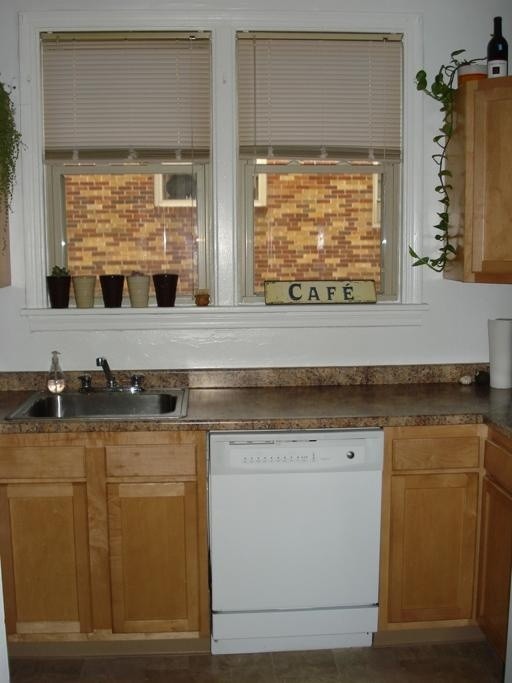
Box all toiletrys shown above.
[48,348,66,393]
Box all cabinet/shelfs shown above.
[383,425,485,629]
[1,164,14,290]
[479,425,511,668]
[0,433,99,643]
[99,431,211,642]
[442,75,511,285]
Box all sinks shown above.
[4,384,191,421]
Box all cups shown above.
[127,275,151,307]
[46,274,69,308]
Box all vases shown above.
[195,293,210,306]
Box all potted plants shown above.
[46,264,71,308]
[126,270,150,308]
[408,48,487,281]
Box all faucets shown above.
[94,355,117,388]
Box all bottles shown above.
[488,14,508,78]
